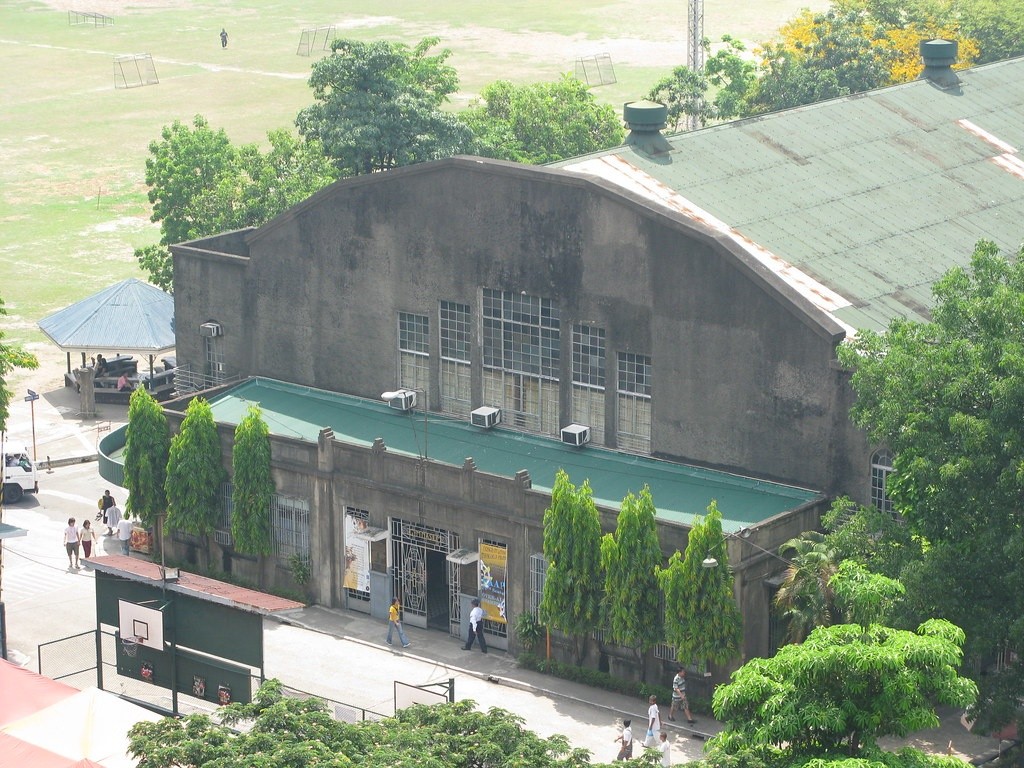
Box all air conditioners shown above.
[389,389,417,410]
[200,323,222,338]
[470,406,501,428]
[560,424,591,446]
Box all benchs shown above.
[107,366,136,377]
[153,367,164,373]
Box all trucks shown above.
[2,440,38,505]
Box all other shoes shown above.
[668,716,676,721]
[386,641,392,646]
[403,642,411,648]
[688,719,697,723]
[462,646,470,650]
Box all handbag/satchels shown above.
[621,739,632,757]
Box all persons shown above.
[614,719,633,762]
[640,695,662,748]
[653,731,670,768]
[91,353,110,387]
[7,453,22,467]
[460,599,488,656]
[98,489,116,536]
[63,517,80,570]
[667,667,698,723]
[385,596,411,648]
[219,28,229,48]
[78,520,97,558]
[117,370,134,391]
[116,513,133,557]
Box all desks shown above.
[80,354,133,370]
